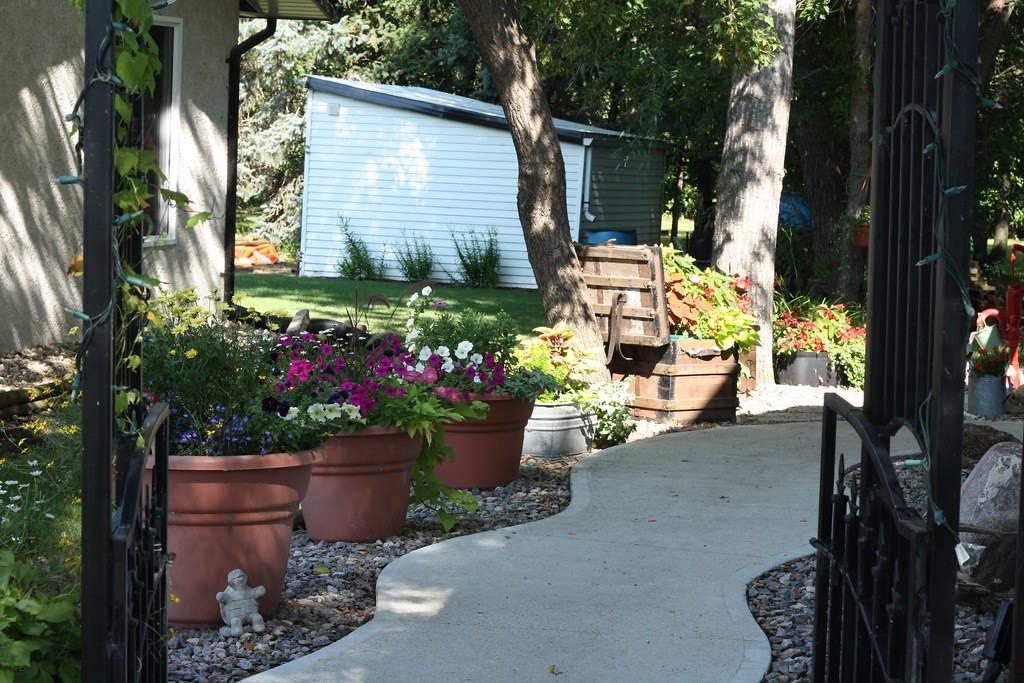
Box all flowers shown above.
[829,205,870,256]
[974,343,1011,378]
[772,301,865,391]
[140,283,637,544]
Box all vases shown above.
[299,425,423,543]
[434,391,536,490]
[851,225,869,247]
[968,375,1007,418]
[115,450,328,630]
[522,401,599,457]
[774,352,847,387]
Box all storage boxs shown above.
[573,247,740,425]
